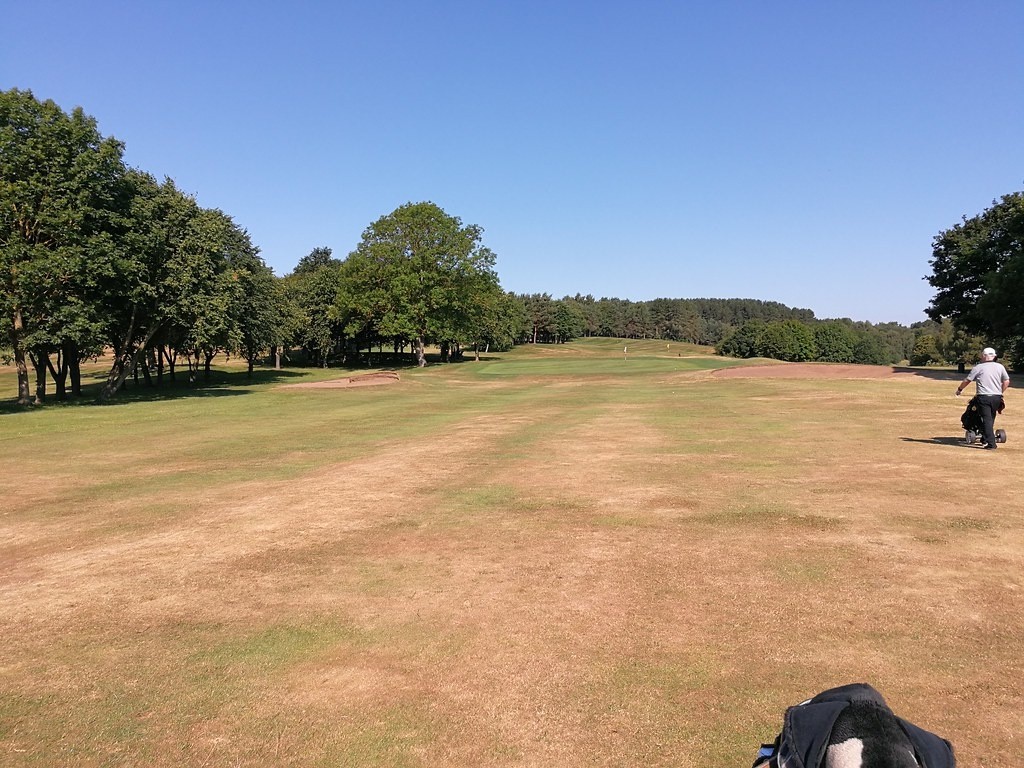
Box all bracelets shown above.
[958,387,962,392]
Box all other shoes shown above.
[980,439,987,444]
[985,445,996,449]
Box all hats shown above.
[983,347,996,356]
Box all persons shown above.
[955,347,1010,450]
